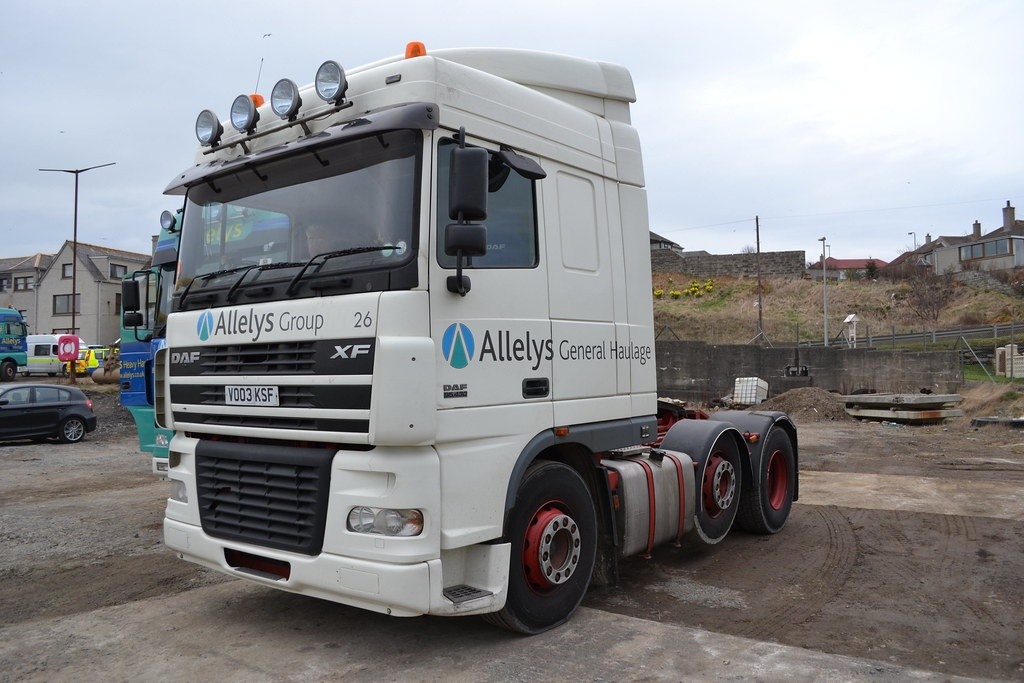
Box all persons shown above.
[299,223,336,262]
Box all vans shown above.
[15,334,89,377]
[66,348,119,376]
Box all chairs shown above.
[9,392,24,404]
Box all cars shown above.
[0,384,97,445]
[87,344,106,349]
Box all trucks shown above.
[0,305,27,381]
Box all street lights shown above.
[908,232,916,251]
[818,236,830,347]
[38,162,117,384]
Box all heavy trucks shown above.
[149,43,801,638]
